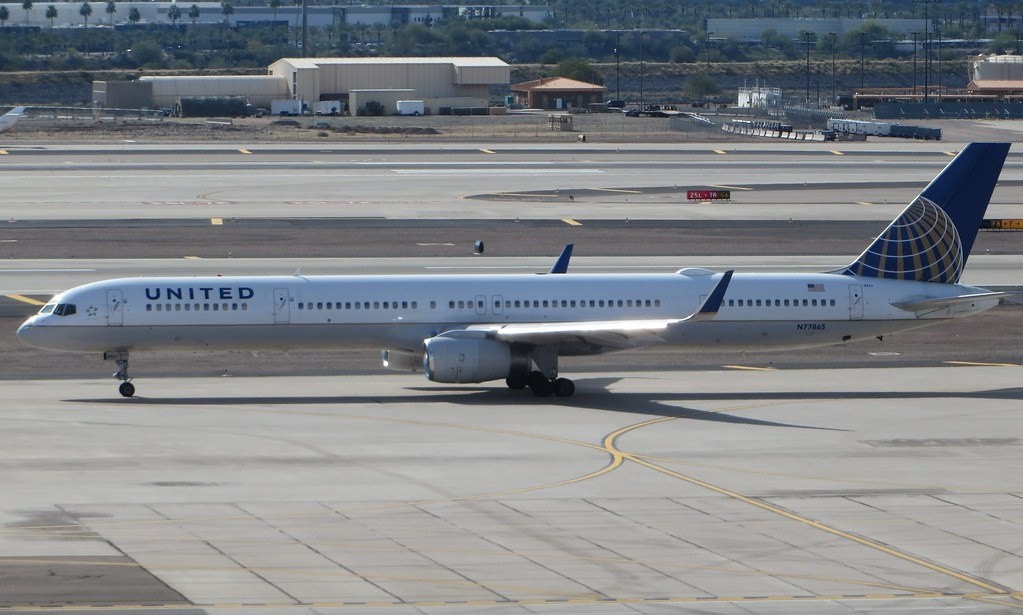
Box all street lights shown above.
[804,32,815,102]
[927,31,934,94]
[708,32,714,76]
[910,31,920,94]
[857,32,866,95]
[640,30,649,109]
[618,33,623,101]
[829,32,836,104]
[935,33,942,101]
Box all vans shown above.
[606,100,626,107]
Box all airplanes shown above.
[14,142,1023,401]
